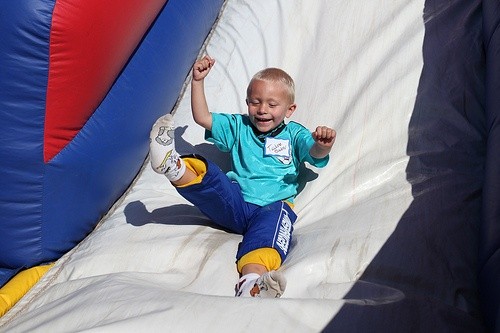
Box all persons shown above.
[148,53,338,300]
